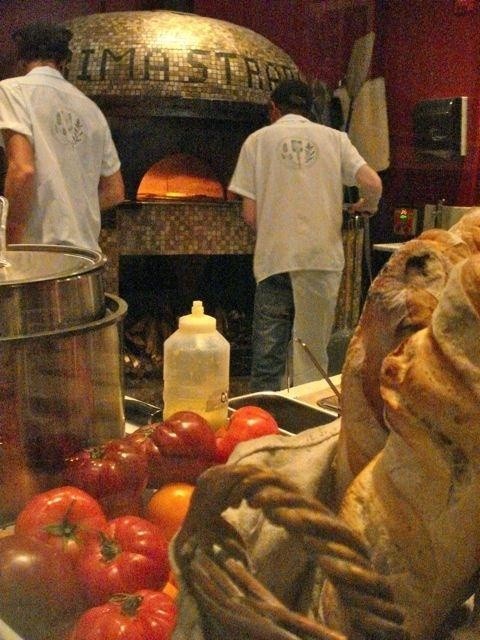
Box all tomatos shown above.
[217,406,279,463]
[0,410,217,640]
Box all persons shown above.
[1,18,127,258]
[227,79,383,391]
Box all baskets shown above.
[173,465,479,637]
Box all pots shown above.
[1,198,128,530]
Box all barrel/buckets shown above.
[2,238,135,522]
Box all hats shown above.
[11,27,73,62]
[271,80,312,109]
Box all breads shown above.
[300,208,480,640]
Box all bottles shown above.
[162,300,229,427]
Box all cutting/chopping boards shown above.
[341,32,376,133]
